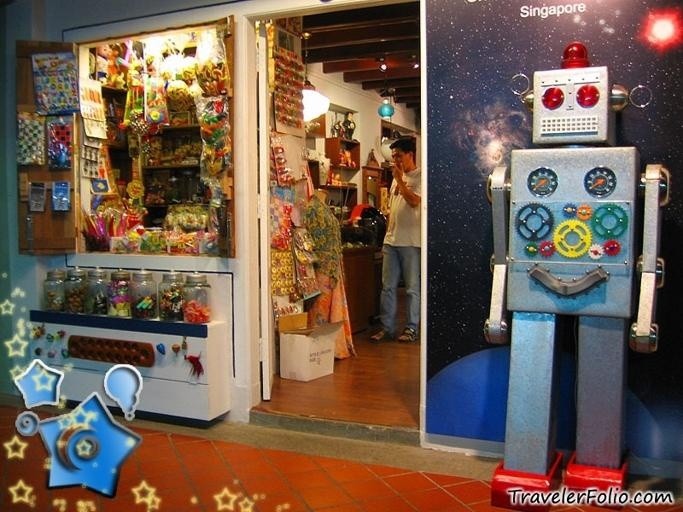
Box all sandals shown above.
[368,328,394,344]
[397,328,419,342]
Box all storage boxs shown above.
[278,312,347,383]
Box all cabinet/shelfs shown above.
[27,307,232,427]
[14,17,237,258]
[304,114,361,225]
[338,248,381,332]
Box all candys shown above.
[43,280,211,324]
[136,208,149,220]
[96,205,128,237]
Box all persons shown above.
[369,136,420,344]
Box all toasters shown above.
[83,268,108,316]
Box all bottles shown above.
[104,270,131,317]
[146,171,203,205]
[129,268,157,318]
[157,272,183,322]
[63,267,87,317]
[41,270,66,310]
[181,273,213,325]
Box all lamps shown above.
[301,32,330,122]
[376,75,394,120]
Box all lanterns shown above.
[377,101,395,121]
